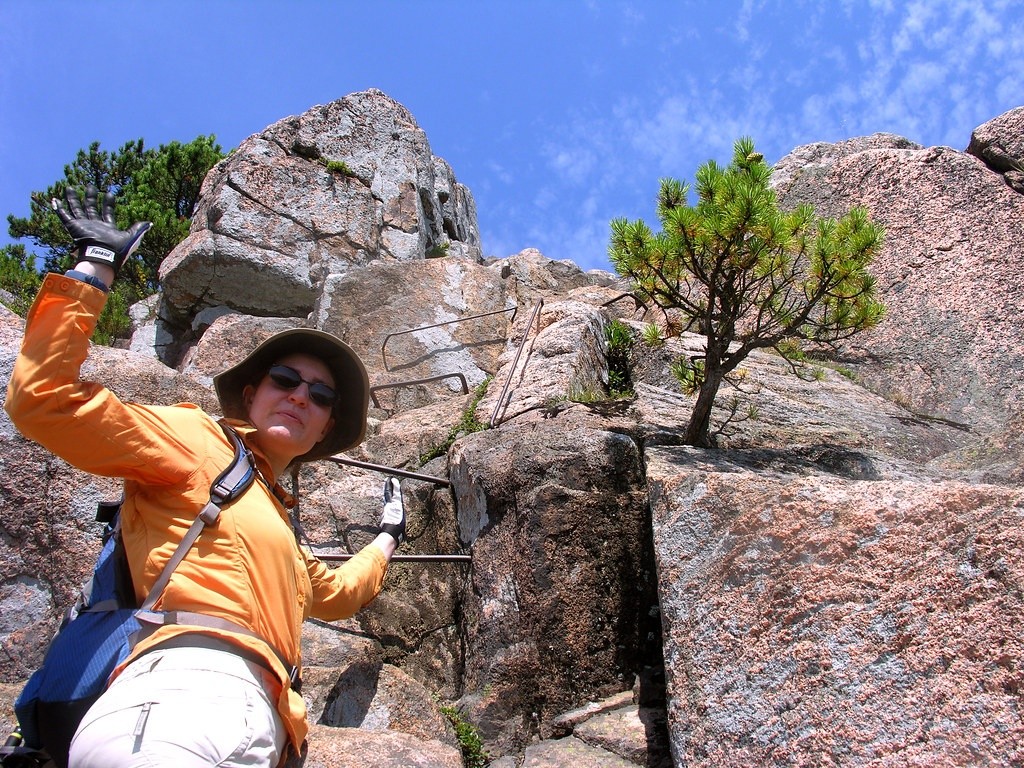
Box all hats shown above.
[211,327,370,462]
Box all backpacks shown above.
[14,417,256,759]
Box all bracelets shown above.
[64,269,110,293]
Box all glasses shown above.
[260,364,339,416]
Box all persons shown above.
[3,186,407,768]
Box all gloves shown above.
[379,477,406,549]
[51,186,154,277]
